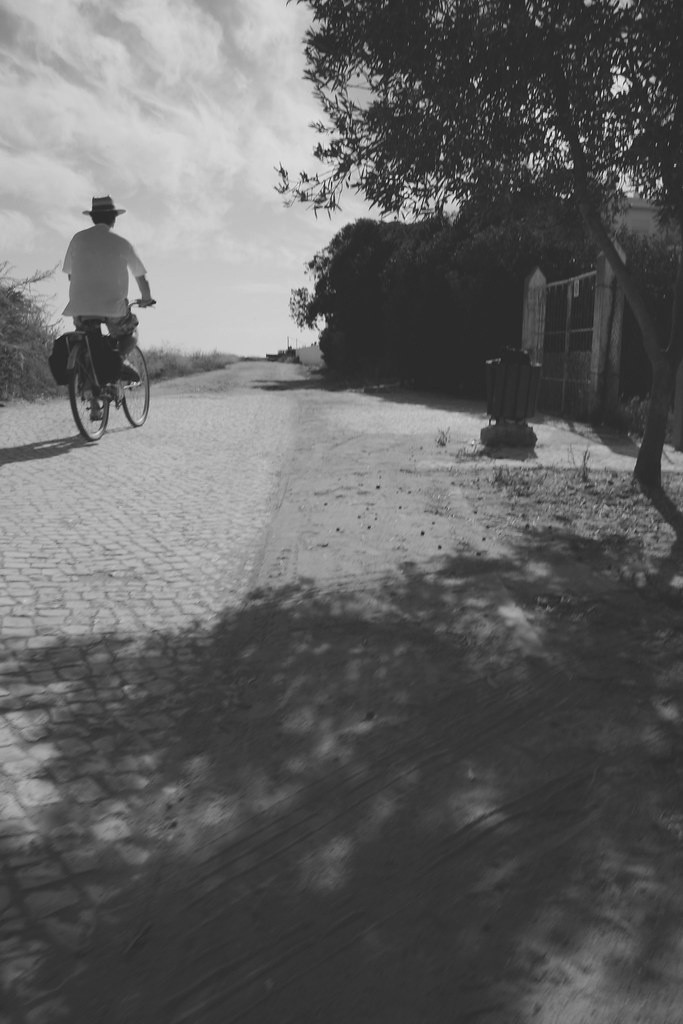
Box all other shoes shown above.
[111,361,139,382]
[89,409,103,420]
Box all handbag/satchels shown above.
[49,334,82,385]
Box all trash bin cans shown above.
[482,347,543,425]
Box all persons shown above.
[63,196,151,421]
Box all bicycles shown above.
[67,299,156,441]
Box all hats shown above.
[82,196,126,216]
